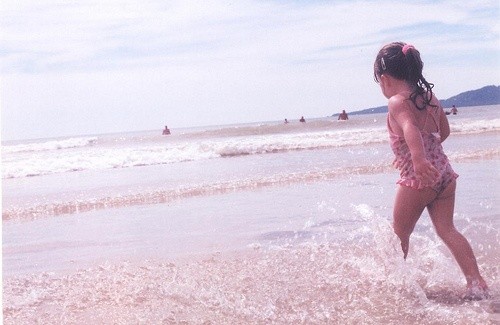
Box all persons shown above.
[372,42,487,289]
[161,125,170,135]
[299,116,305,122]
[451,104,458,114]
[338,110,349,121]
